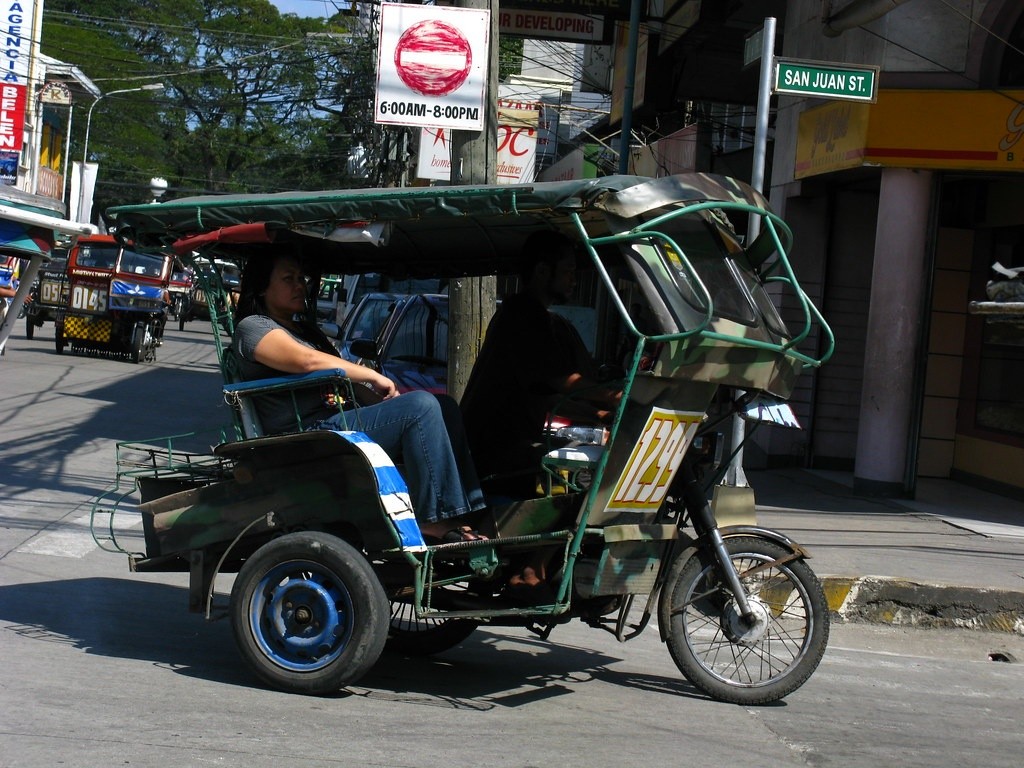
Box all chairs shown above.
[220,349,357,468]
[142,261,159,277]
[95,257,110,267]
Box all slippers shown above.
[423,522,484,546]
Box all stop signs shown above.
[373,3,490,134]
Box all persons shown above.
[0,286,33,304]
[459,231,625,605]
[141,262,171,346]
[232,252,491,543]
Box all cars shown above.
[348,292,502,400]
[321,293,414,362]
[317,278,344,322]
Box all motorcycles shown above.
[22,241,82,341]
[56,232,173,363]
[86,171,835,707]
[169,256,241,332]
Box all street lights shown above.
[76,83,165,221]
